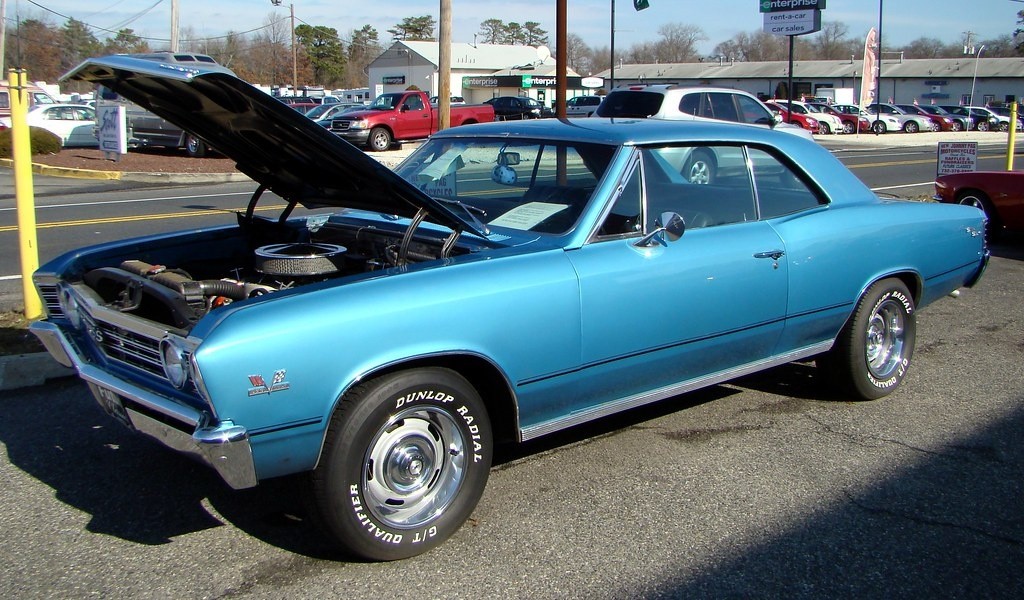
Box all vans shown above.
[91,53,238,157]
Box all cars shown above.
[850,103,934,134]
[30,53,991,562]
[918,104,974,131]
[739,102,820,133]
[276,97,342,119]
[0,86,61,116]
[316,105,387,128]
[550,95,607,117]
[305,103,363,122]
[987,107,1024,124]
[483,96,555,120]
[428,96,466,109]
[61,100,96,120]
[894,104,953,132]
[820,104,903,134]
[789,104,869,134]
[954,107,1022,132]
[932,169,1024,244]
[751,99,844,135]
[939,105,999,131]
[0,104,100,147]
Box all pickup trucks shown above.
[330,90,494,152]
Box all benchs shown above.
[618,182,819,224]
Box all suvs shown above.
[588,82,816,189]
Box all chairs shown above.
[519,180,588,216]
[619,204,716,233]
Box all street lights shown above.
[272,0,297,97]
